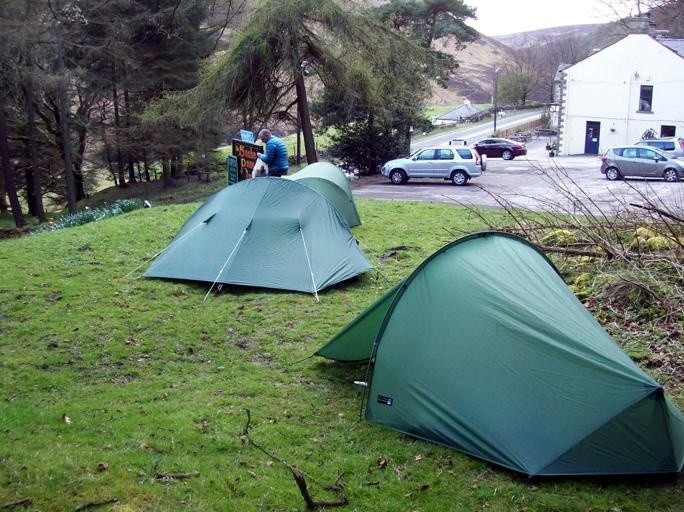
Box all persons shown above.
[255,129,289,178]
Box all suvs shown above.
[379,142,489,187]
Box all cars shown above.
[598,146,684,182]
[470,136,529,161]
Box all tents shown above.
[308,226,683,479]
[290,160,362,227]
[140,175,374,295]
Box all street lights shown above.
[493,67,500,132]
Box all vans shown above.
[631,135,684,163]
